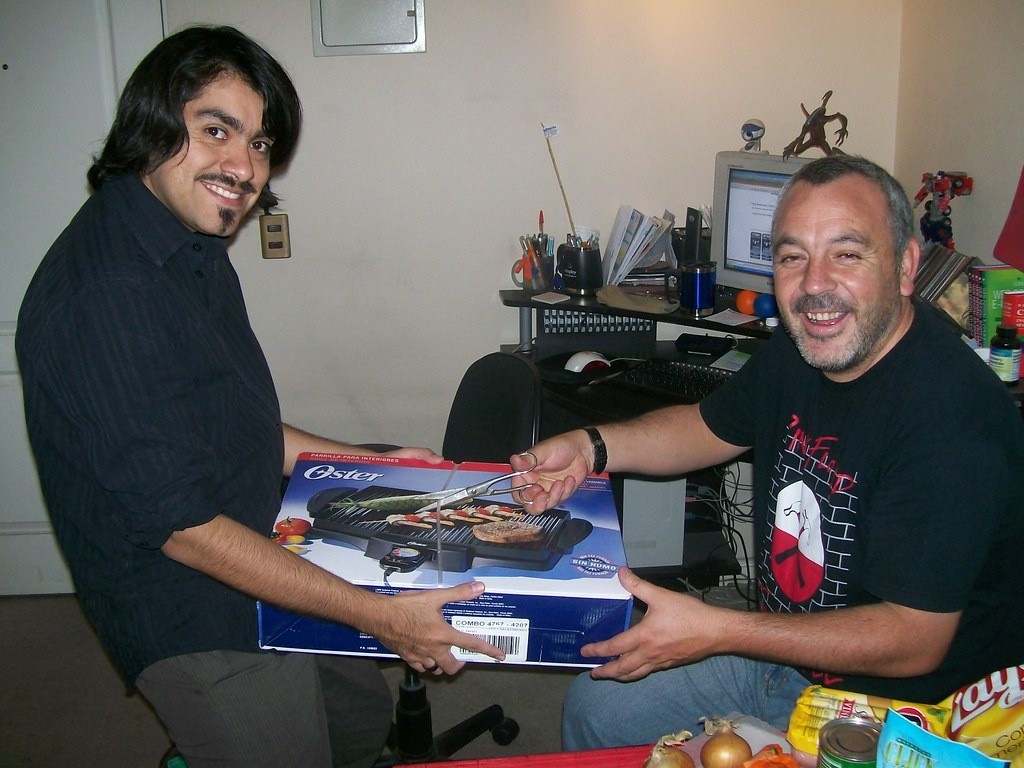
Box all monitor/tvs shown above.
[710,151,819,296]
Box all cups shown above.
[680,260,717,318]
[511,255,554,294]
[559,244,603,294]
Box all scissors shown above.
[409,452,541,514]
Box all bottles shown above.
[989,324,1023,388]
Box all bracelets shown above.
[583,425,607,475]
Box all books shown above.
[601,204,672,288]
[966,264,1024,349]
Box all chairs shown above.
[344,351,540,768]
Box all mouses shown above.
[563,352,611,373]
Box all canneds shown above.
[815,717,883,768]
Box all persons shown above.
[510,154,1024,754]
[14,25,506,768]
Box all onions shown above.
[642,716,752,768]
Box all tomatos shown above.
[270,516,312,555]
[405,506,512,522]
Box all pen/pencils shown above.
[567,232,600,250]
[539,209,544,231]
[519,233,554,291]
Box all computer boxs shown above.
[622,473,686,569]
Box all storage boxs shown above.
[257,451,632,670]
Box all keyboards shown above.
[611,357,740,403]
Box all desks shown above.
[393,730,810,768]
[499,284,1017,589]
